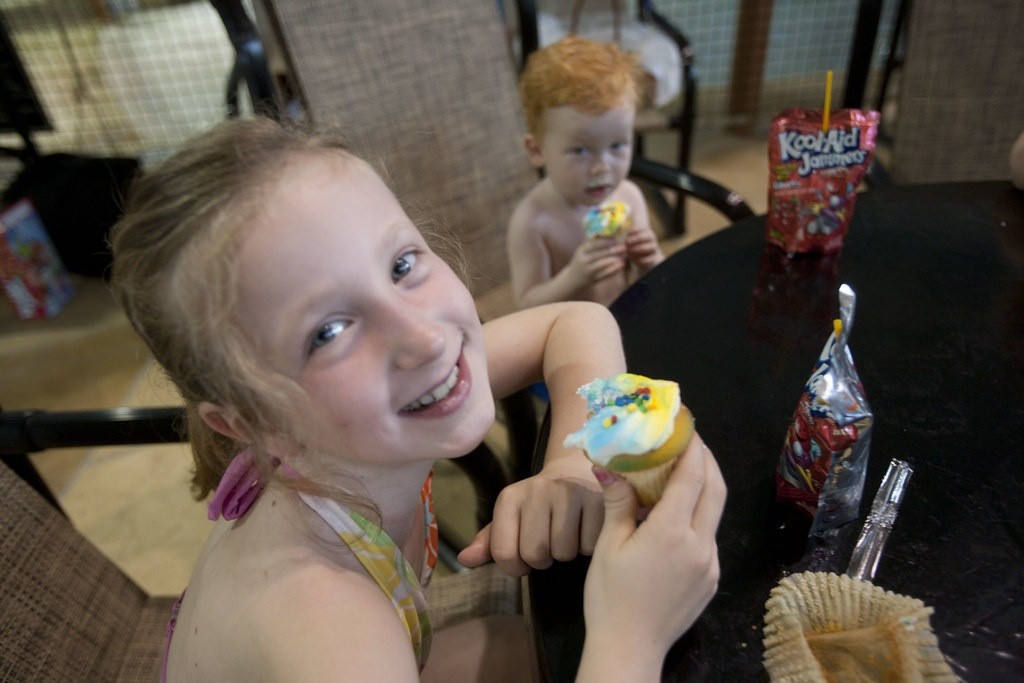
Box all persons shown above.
[505,36,669,309]
[111,119,728,683]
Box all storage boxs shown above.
[0,198,76,325]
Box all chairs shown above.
[518,0,700,239]
[0,405,514,683]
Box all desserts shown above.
[583,205,636,251]
[564,375,696,510]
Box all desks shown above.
[526,179,1024,683]
